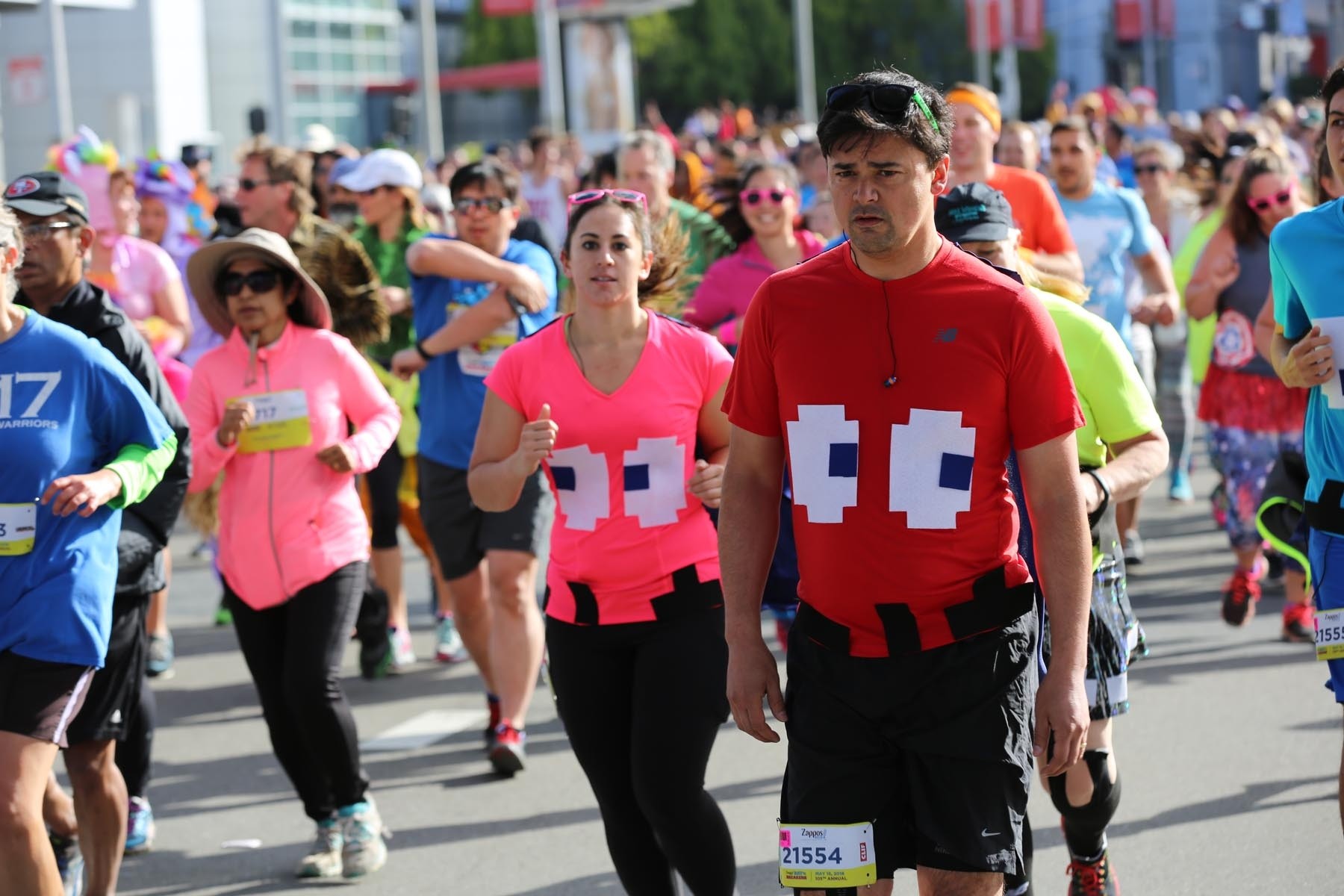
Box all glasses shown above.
[1244,178,1293,212]
[567,188,649,235]
[22,222,74,248]
[455,195,512,215]
[739,189,798,206]
[217,268,280,295]
[1134,164,1166,174]
[240,178,275,191]
[825,83,941,138]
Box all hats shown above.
[3,171,88,223]
[185,226,333,339]
[182,145,212,168]
[933,182,1015,243]
[336,149,422,193]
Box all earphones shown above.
[842,225,901,392]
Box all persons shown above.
[0,60,1344,896]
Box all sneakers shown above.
[1065,816,1119,895]
[386,624,418,673]
[1283,602,1316,645]
[490,719,524,775]
[49,830,85,896]
[216,594,232,626]
[294,810,344,877]
[1123,528,1143,565]
[1170,469,1191,501]
[436,612,468,662]
[484,693,501,743]
[1222,566,1261,626]
[338,789,391,879]
[123,795,155,856]
[145,624,174,673]
